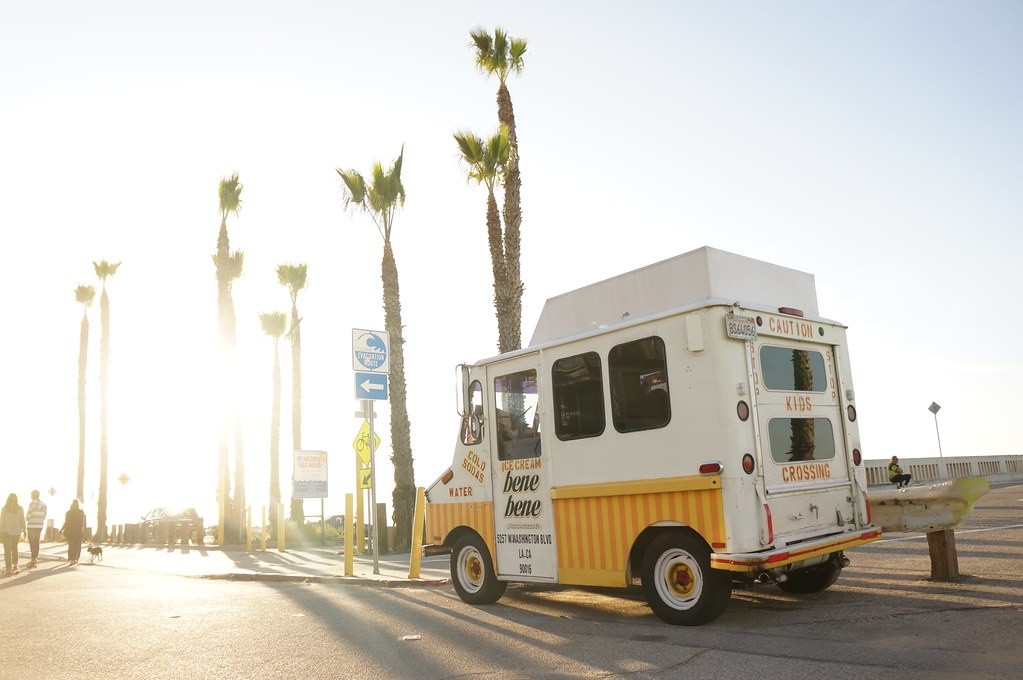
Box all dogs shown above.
[86,544,103,561]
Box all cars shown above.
[205,526,217,535]
[137,506,201,544]
[325,515,372,537]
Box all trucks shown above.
[421,247,885,628]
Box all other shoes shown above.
[27,558,38,568]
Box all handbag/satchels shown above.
[81,527,92,541]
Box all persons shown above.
[59,500,87,564]
[26,491,47,568]
[888,456,911,489]
[0,493,27,574]
